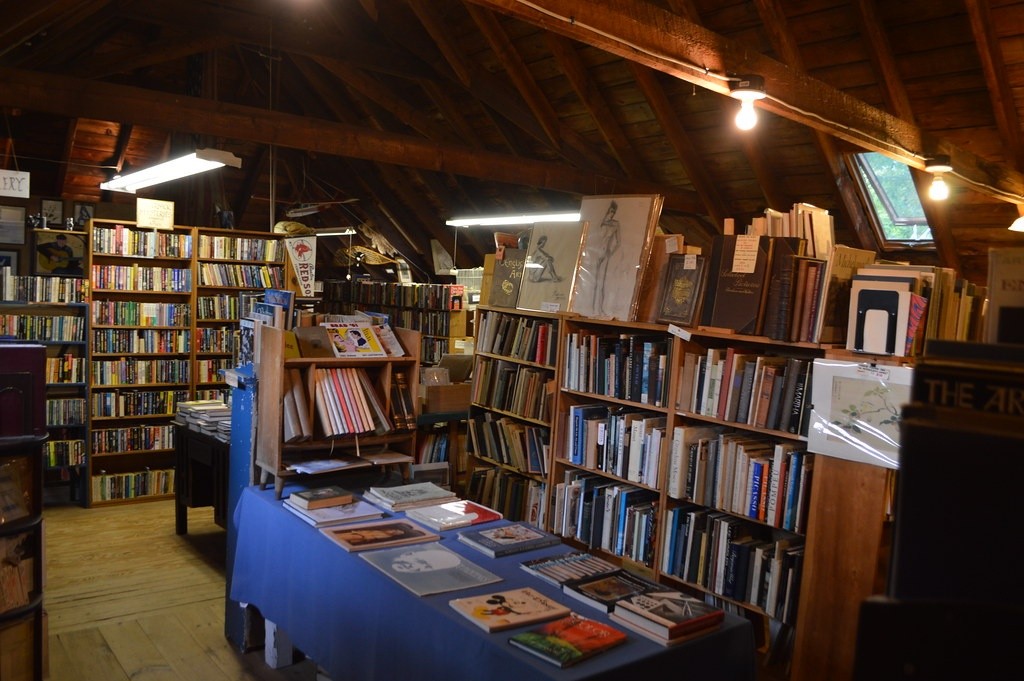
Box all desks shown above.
[243,469,751,681]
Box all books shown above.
[194,357,232,404]
[195,290,265,352]
[46,398,87,425]
[91,463,176,502]
[92,262,192,293]
[236,287,301,367]
[0,267,88,342]
[319,276,465,366]
[196,234,287,288]
[91,356,192,384]
[92,300,191,327]
[282,306,505,596]
[45,352,88,384]
[44,438,86,466]
[93,388,191,416]
[407,200,994,638]
[171,399,231,444]
[93,328,191,354]
[93,224,193,258]
[91,424,174,454]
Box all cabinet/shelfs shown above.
[0,191,1024,681]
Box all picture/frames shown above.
[807,358,914,471]
[72,201,97,230]
[655,253,710,329]
[30,228,90,279]
[40,197,65,229]
[0,204,27,246]
[0,249,21,276]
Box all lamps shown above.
[100,148,242,194]
[315,226,357,236]
[445,210,581,227]
